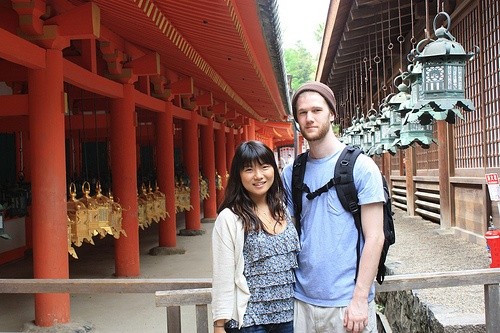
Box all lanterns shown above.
[340,37,476,157]
[68,170,230,259]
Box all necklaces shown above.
[258,208,273,224]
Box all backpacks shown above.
[292,146,395,285]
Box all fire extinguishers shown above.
[484,214,500,269]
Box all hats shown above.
[292,81,336,122]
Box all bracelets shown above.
[213,325,225,327]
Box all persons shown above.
[279,81,386,333]
[211,141,302,333]
[287,154,292,165]
[280,157,285,172]
